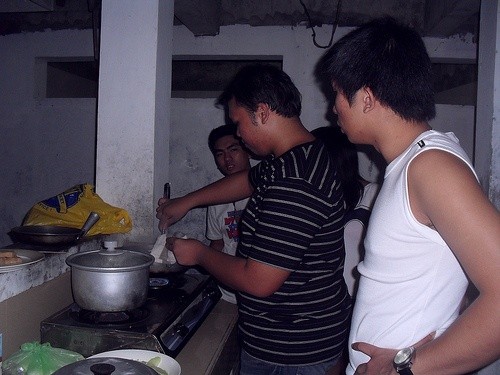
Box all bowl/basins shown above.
[88,350,181,375]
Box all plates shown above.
[0,249,45,273]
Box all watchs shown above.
[392,345,414,375]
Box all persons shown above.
[156,61,379,375]
[328,17,500,375]
[206,125,257,305]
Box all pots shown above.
[7,211,100,252]
[65,238,156,313]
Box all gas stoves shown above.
[40,269,223,359]
